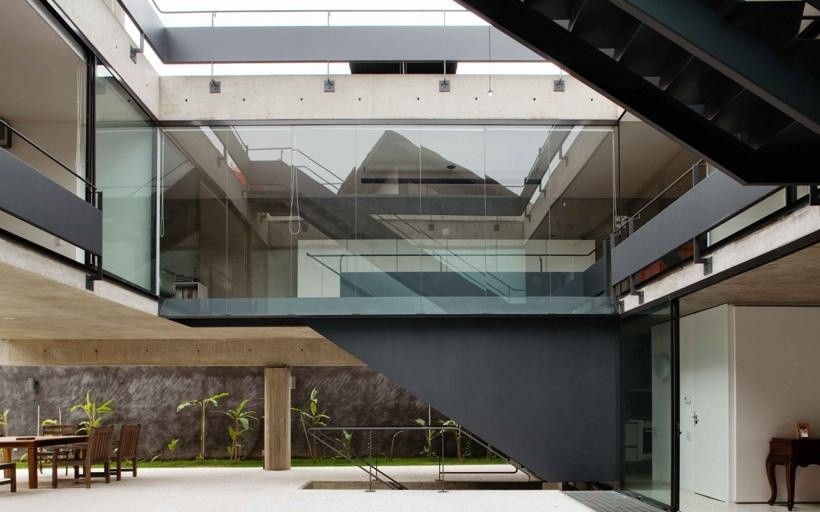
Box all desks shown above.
[0,435,92,489]
[764,437,820,511]
[172,282,208,300]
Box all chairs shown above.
[43,421,141,489]
[0,463,17,492]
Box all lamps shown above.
[29,378,41,391]
